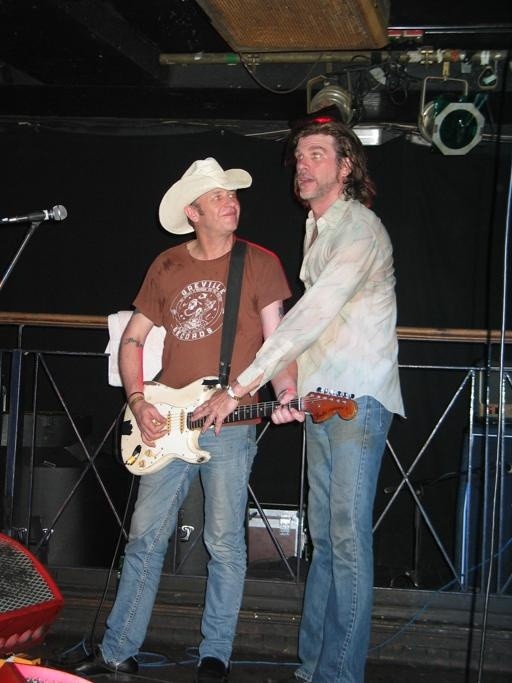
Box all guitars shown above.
[120,376,359,476]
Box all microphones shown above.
[0,205,68,225]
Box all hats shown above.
[158,156,253,235]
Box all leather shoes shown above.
[72,646,139,680]
[196,657,230,683]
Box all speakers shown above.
[0,533,65,658]
